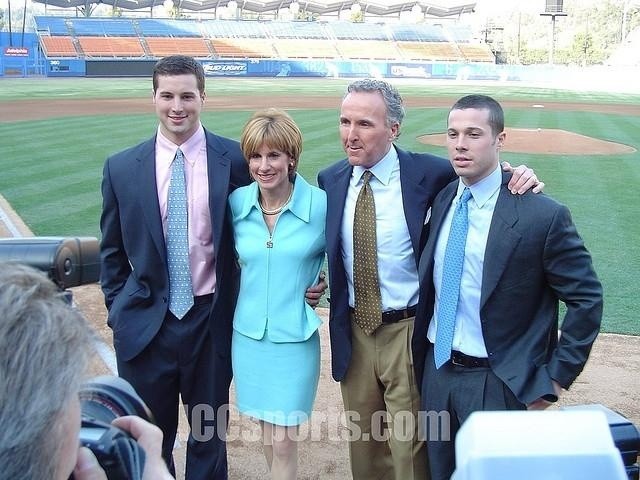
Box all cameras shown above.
[78,375,157,480]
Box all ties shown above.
[433,189,475,371]
[165,150,195,320]
[352,170,383,337]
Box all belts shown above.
[431,342,490,369]
[346,304,418,326]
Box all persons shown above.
[413,93,605,480]
[314,77,546,480]
[95,51,329,480]
[2,262,177,480]
[223,107,327,480]
